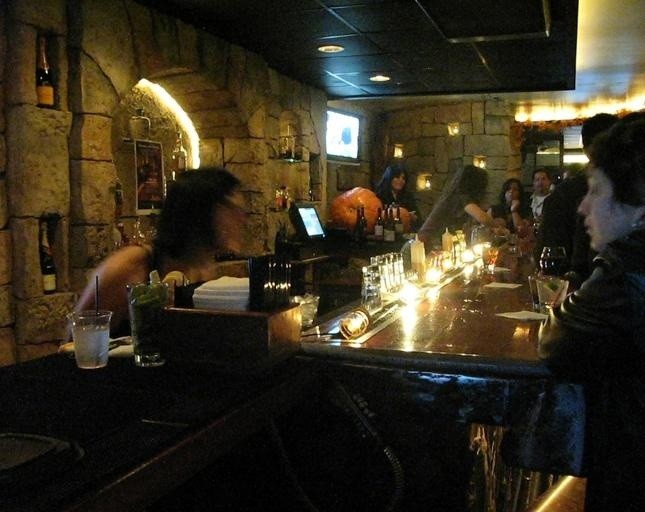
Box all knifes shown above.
[68,334,132,360]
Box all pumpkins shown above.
[331,186,382,233]
[381,208,411,232]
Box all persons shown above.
[396,164,506,270]
[375,161,424,229]
[55,163,246,343]
[529,111,645,512]
[530,169,556,220]
[536,112,620,477]
[479,176,536,238]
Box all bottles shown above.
[34,37,54,109]
[111,221,156,249]
[442,227,467,251]
[114,178,124,216]
[172,132,187,174]
[471,224,485,243]
[410,233,426,271]
[38,221,57,295]
[360,252,404,311]
[286,123,295,159]
[355,204,405,243]
[275,183,289,210]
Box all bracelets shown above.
[510,209,520,213]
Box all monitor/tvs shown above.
[326,105,363,167]
[288,202,327,242]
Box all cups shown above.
[124,280,170,367]
[65,309,112,369]
[528,274,570,310]
[482,246,499,276]
[507,233,518,246]
[291,294,321,328]
[539,246,568,274]
